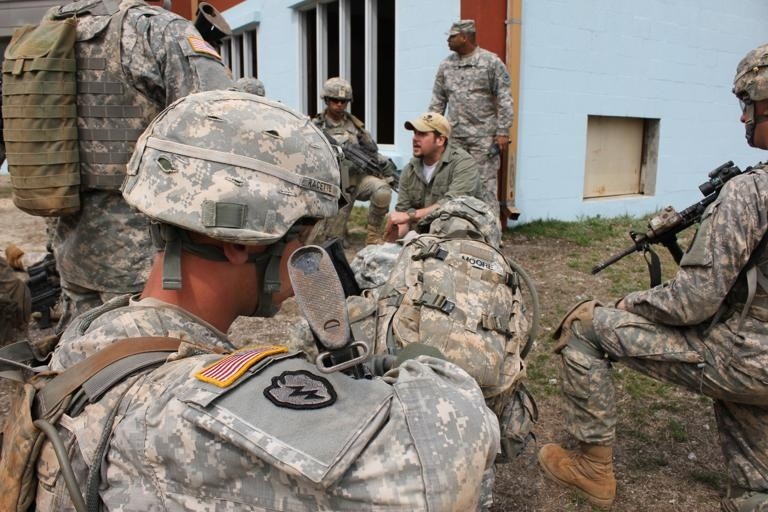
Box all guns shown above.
[285,242,374,388]
[590,163,752,276]
[190,1,232,46]
[323,130,400,192]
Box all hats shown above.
[404,112,452,139]
[445,19,476,36]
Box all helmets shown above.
[320,77,354,103]
[118,87,341,247]
[731,42,768,101]
[416,194,503,250]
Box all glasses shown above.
[285,218,319,244]
[330,98,346,104]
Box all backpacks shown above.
[383,231,525,400]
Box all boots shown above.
[364,224,383,247]
[538,441,617,507]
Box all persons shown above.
[234,77,267,97]
[538,43,768,512]
[430,15,514,248]
[0,0,230,315]
[353,195,541,468]
[1,93,501,511]
[386,112,484,237]
[310,78,393,245]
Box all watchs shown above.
[406,208,416,223]
